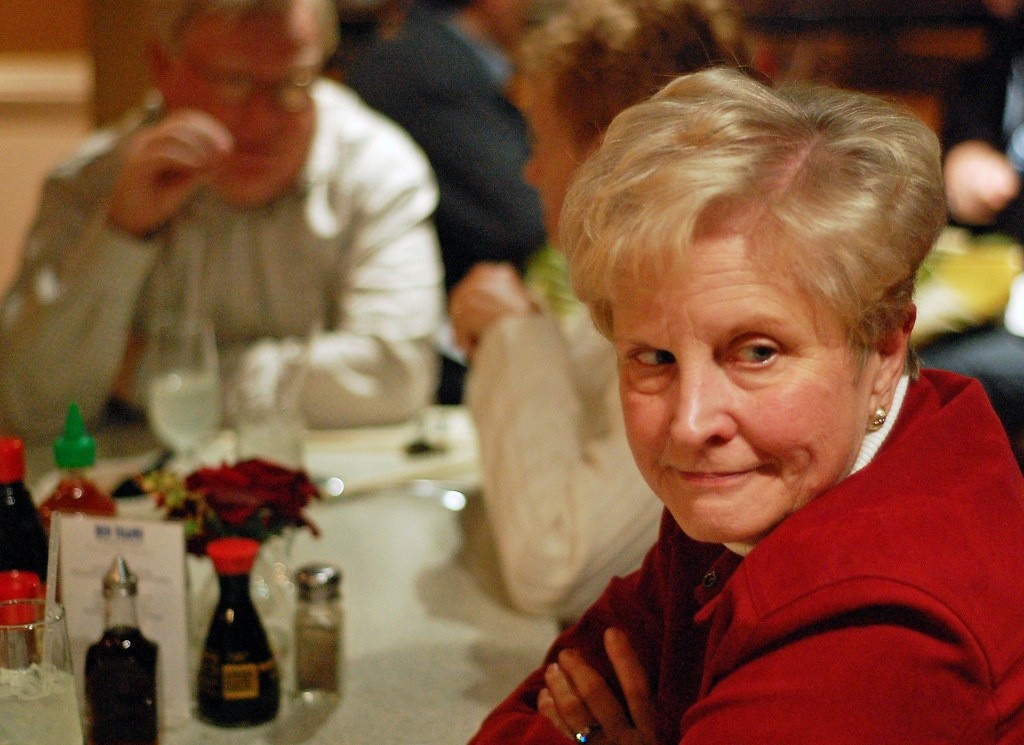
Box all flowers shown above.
[138,460,322,550]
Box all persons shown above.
[0,0,445,429]
[939,44,1024,243]
[448,0,742,621]
[466,67,1024,745]
[321,0,545,405]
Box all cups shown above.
[146,316,223,450]
[0,598,84,745]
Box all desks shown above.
[0,404,628,745]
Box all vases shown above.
[186,526,298,664]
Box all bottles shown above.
[81,556,165,745]
[293,561,345,705]
[0,434,49,641]
[37,401,118,535]
[194,538,282,729]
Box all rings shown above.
[574,724,600,745]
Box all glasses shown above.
[156,37,325,114]
